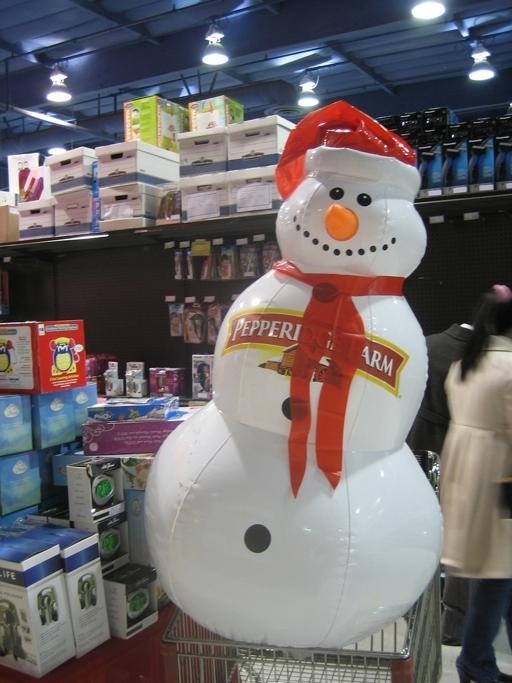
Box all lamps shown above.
[49,64,68,81]
[411,0,445,20]
[297,87,319,107]
[468,59,495,81]
[299,71,315,87]
[204,23,224,40]
[46,82,71,102]
[202,40,229,65]
[471,40,491,59]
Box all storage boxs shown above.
[0,452,42,516]
[0,533,77,679]
[0,320,88,394]
[81,407,201,456]
[31,390,76,450]
[0,394,33,457]
[51,447,153,491]
[70,381,98,437]
[10,524,111,659]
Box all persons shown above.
[217,255,231,279]
[408,323,484,648]
[439,283,512,683]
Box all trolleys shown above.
[161,559,448,678]
